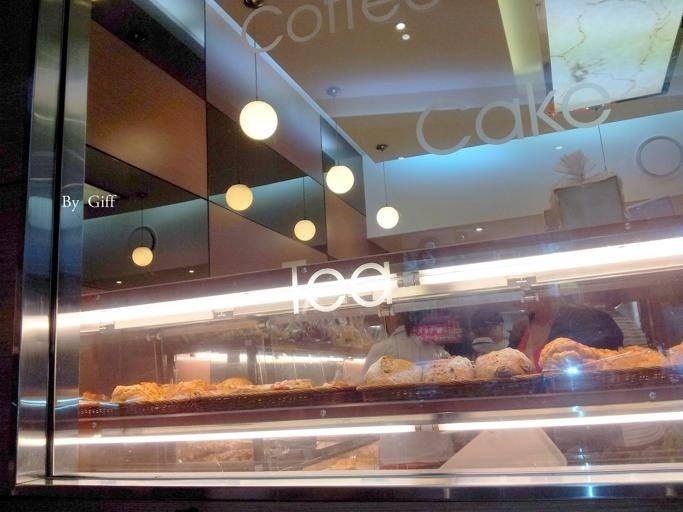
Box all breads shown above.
[80,336,683,406]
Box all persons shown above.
[361,295,669,473]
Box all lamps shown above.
[131,192,154,267]
[294,173,317,243]
[81,273,396,324]
[224,142,253,210]
[325,86,355,195]
[375,144,400,230]
[396,237,683,287]
[432,410,683,432]
[78,424,422,446]
[238,0,278,141]
